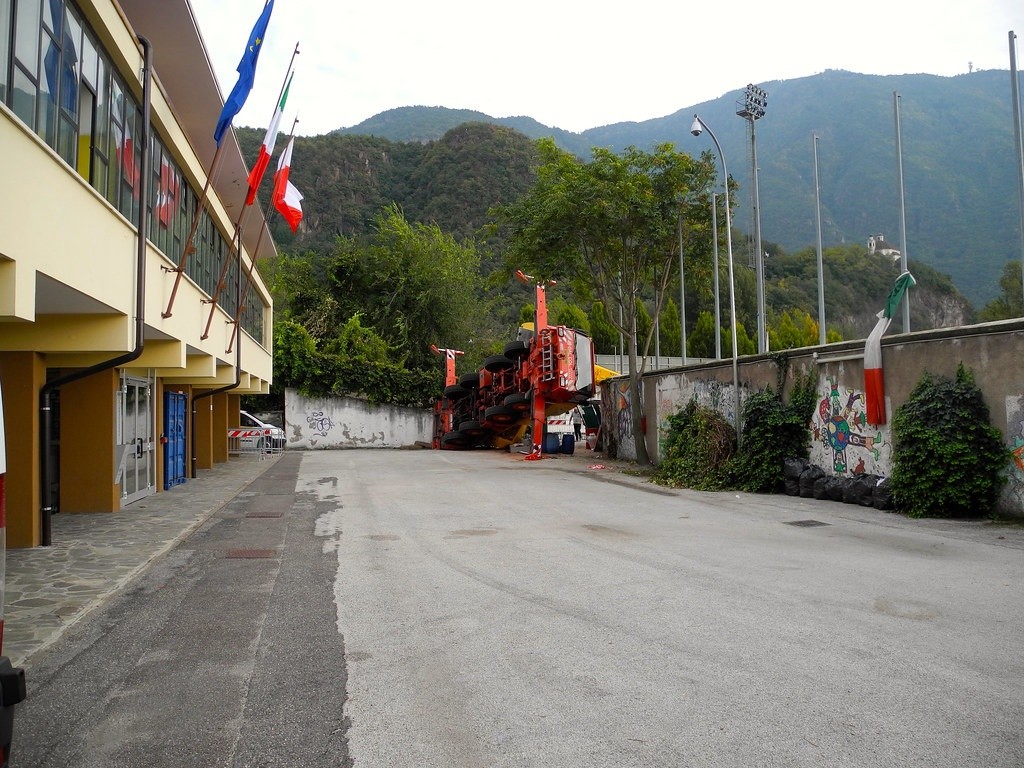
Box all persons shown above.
[569,407,582,442]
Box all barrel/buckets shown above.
[547,432,560,454]
[562,434,575,454]
[586,427,598,449]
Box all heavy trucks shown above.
[429,270,631,461]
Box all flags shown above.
[112,94,140,199]
[214,0,304,236]
[154,153,179,227]
[43,0,81,114]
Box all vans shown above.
[240,410,287,455]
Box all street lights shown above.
[736,84,771,352]
[690,112,741,454]
